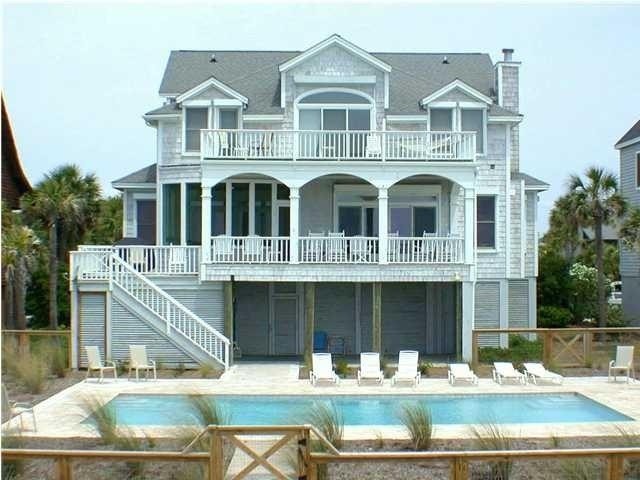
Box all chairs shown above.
[203,130,458,160]
[84,346,118,384]
[524,363,562,386]
[493,362,524,384]
[128,345,157,383]
[1,382,38,433]
[391,350,421,386]
[311,352,341,387]
[357,352,384,385]
[447,363,478,385]
[607,346,635,383]
